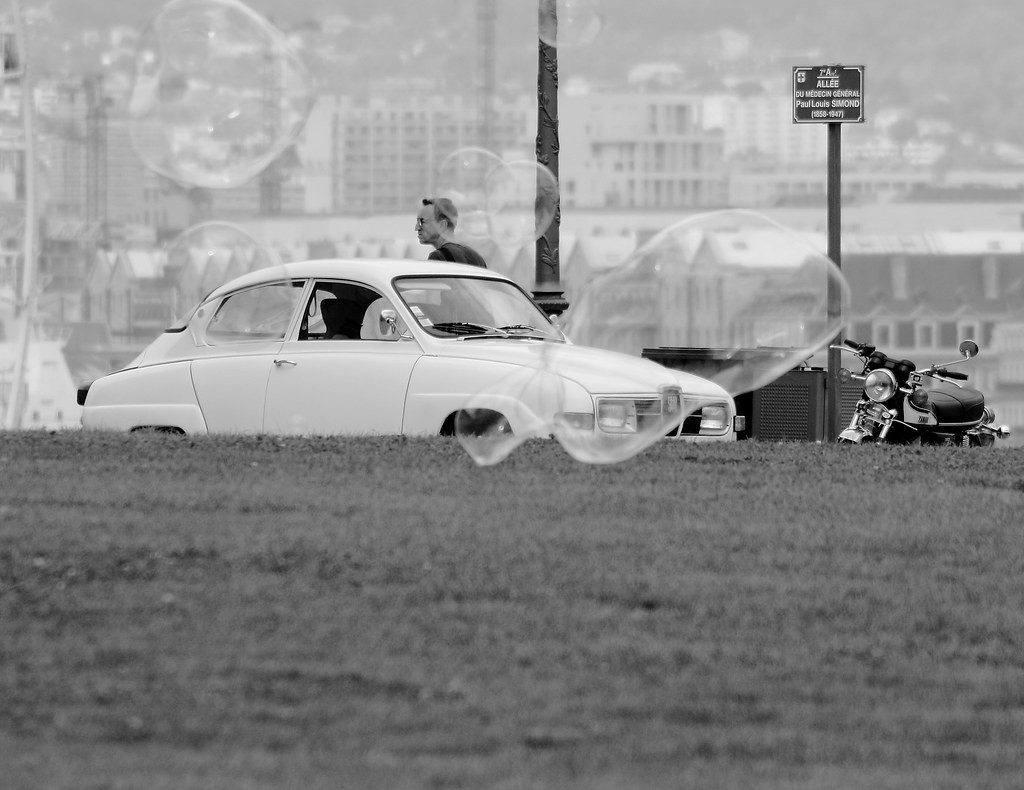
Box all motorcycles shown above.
[828,339,1011,448]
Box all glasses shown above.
[417,217,435,227]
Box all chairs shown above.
[320,298,367,340]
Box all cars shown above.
[76,256,749,449]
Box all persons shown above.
[415,198,487,269]
